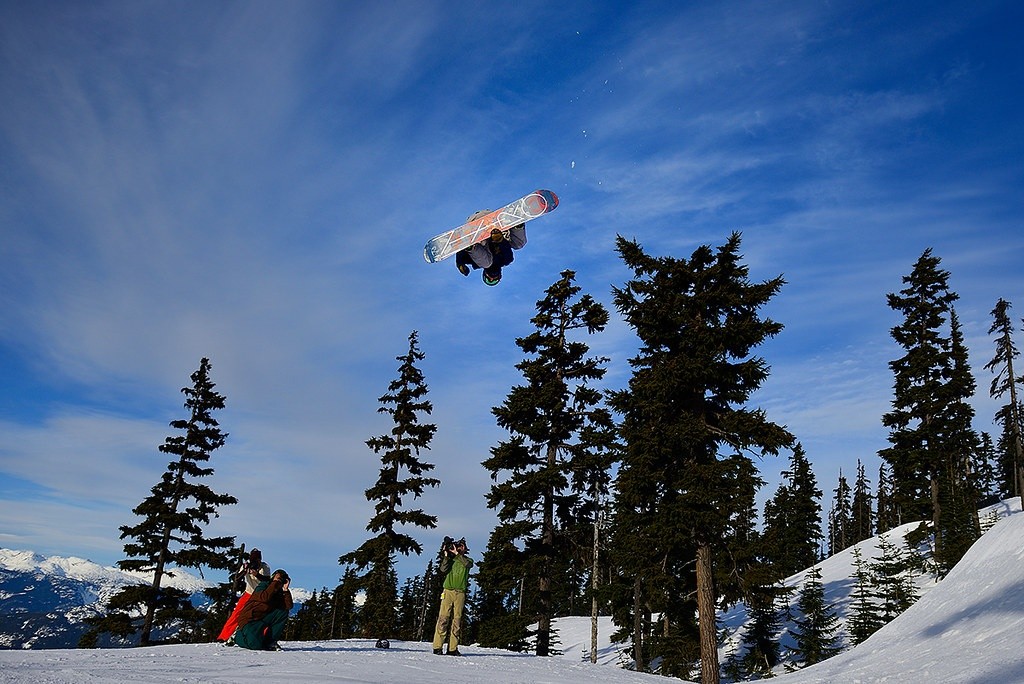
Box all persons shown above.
[456,209,527,286]
[217,549,270,642]
[433,541,473,655]
[226,569,293,651]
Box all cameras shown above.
[279,575,289,583]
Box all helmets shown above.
[483,271,501,287]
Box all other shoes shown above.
[434,649,443,655]
[458,264,469,276]
[465,245,475,253]
[264,639,281,650]
[447,649,462,656]
[515,223,525,230]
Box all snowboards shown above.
[423,189,559,264]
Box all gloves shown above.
[491,229,503,243]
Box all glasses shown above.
[485,278,498,285]
